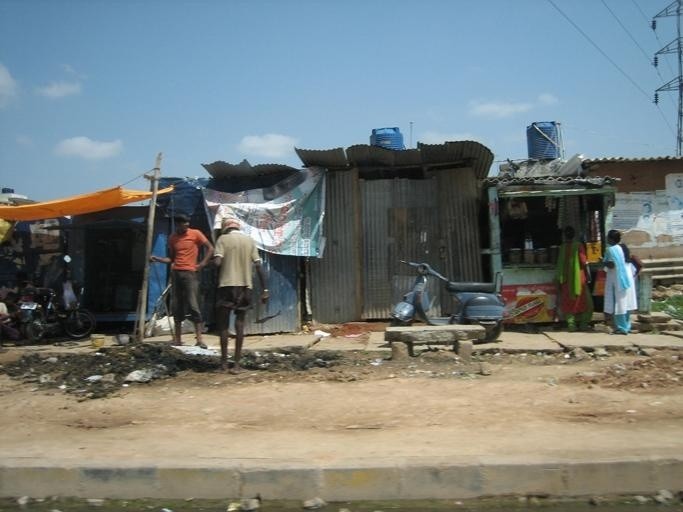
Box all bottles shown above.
[509,246,559,266]
[524,235,533,250]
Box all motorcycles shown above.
[17,256,96,344]
[389,260,505,342]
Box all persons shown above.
[596,229,634,335]
[53,266,85,315]
[552,224,595,332]
[148,212,215,350]
[17,285,45,312]
[204,218,271,375]
[0,291,22,342]
[619,242,642,312]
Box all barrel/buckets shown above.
[370,127,403,151]
[526,122,560,160]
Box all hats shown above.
[219,219,240,233]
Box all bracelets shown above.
[263,289,269,292]
